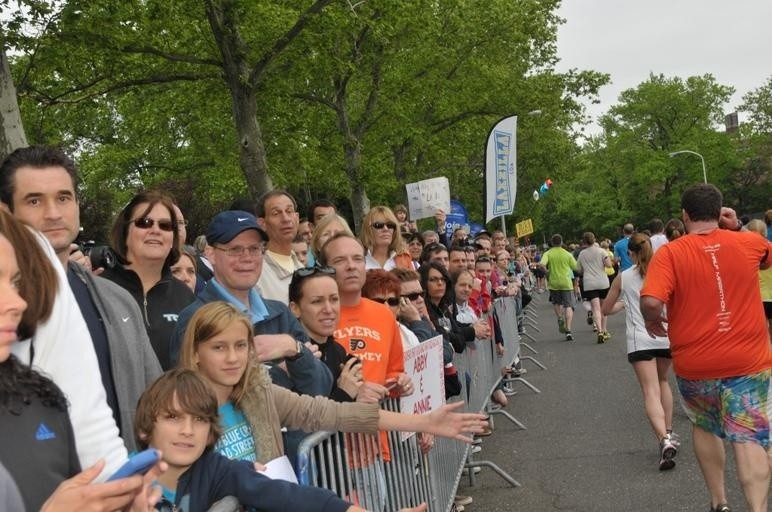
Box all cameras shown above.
[76,232,117,273]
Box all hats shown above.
[206,211,270,246]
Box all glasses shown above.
[296,264,336,278]
[214,245,263,256]
[372,222,395,229]
[401,292,427,301]
[373,297,401,306]
[127,217,175,232]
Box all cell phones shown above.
[106,447,159,483]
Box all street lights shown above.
[667,150,707,184]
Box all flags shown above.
[482,115,518,225]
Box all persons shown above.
[0,145,162,510]
[744,218,771,320]
[640,183,771,512]
[541,232,611,343]
[67,190,538,511]
[601,218,687,471]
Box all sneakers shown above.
[586,310,593,324]
[667,431,681,447]
[597,333,604,344]
[565,329,574,340]
[558,317,565,333]
[593,322,598,332]
[657,439,676,472]
[602,332,612,339]
